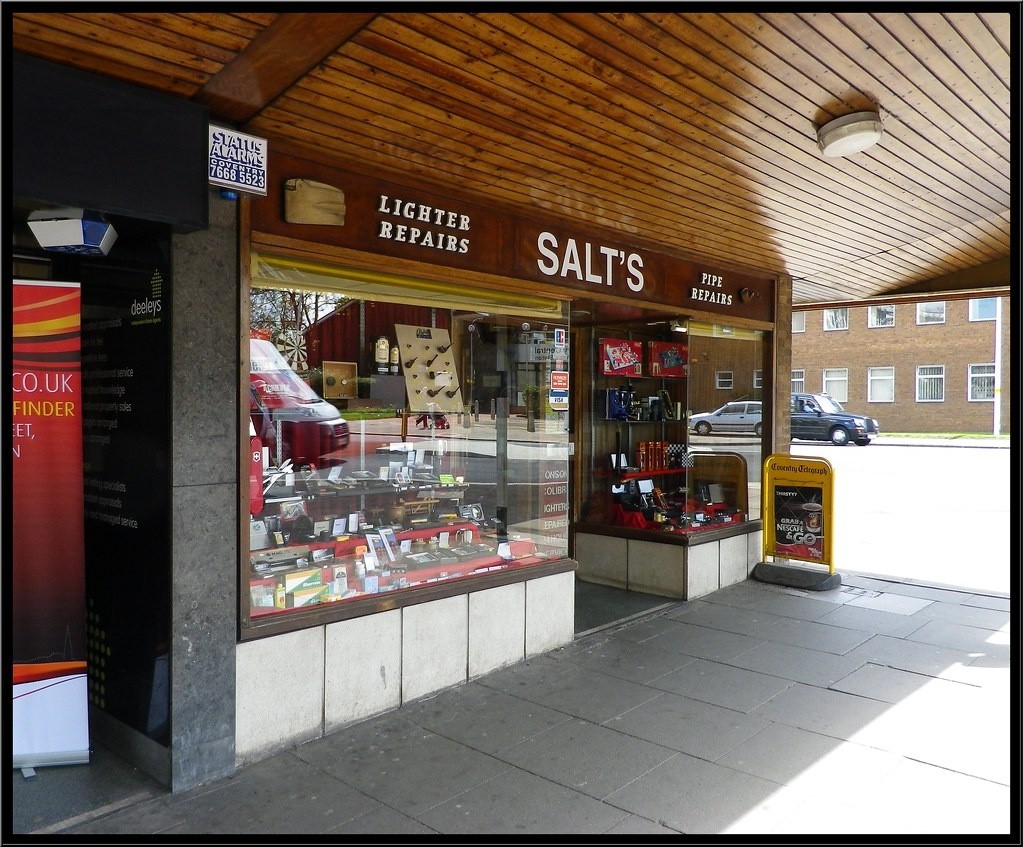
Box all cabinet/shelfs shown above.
[248,413,573,623]
[591,375,688,481]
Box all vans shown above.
[249,339,351,465]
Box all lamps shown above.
[817,110,885,158]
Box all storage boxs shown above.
[648,341,688,377]
[598,338,643,378]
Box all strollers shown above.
[427,401,451,430]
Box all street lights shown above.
[466,324,479,426]
[523,320,535,433]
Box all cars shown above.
[690,400,763,438]
[789,394,880,446]
